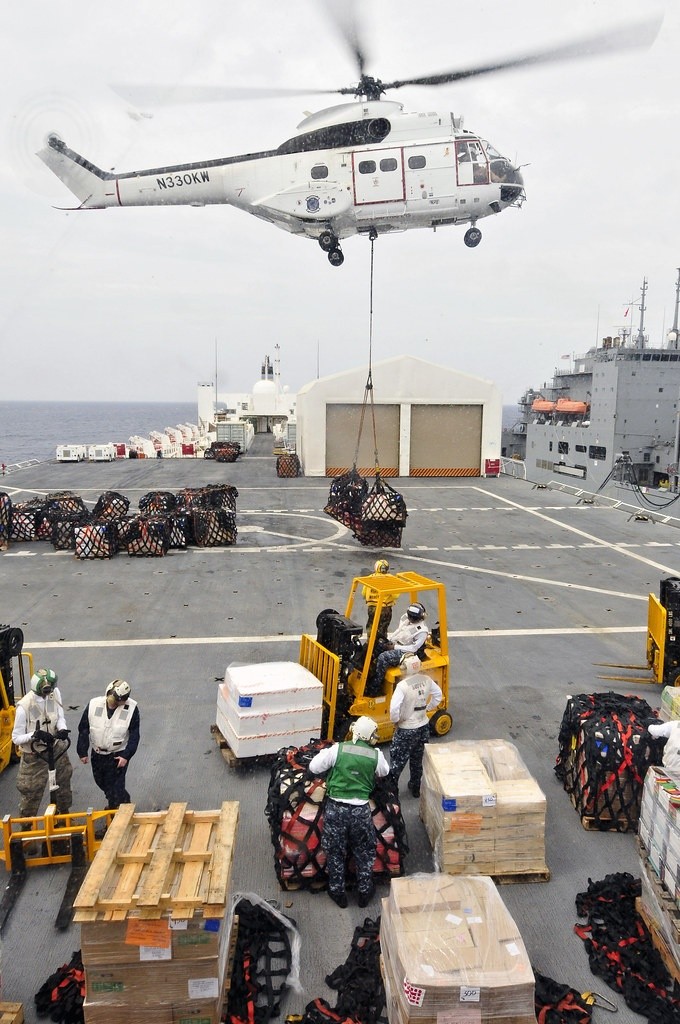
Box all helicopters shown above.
[32,1,662,268]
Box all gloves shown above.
[54,729,71,741]
[34,730,51,746]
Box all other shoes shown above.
[95,828,107,839]
[358,887,376,908]
[407,781,420,798]
[327,887,348,908]
[54,811,79,827]
[22,840,32,850]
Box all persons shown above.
[362,560,400,640]
[460,147,507,182]
[389,651,443,799]
[537,418,545,423]
[11,668,74,831]
[655,478,668,490]
[309,715,389,908]
[367,603,428,697]
[77,679,139,839]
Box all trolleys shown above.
[0,734,119,935]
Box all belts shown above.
[329,799,362,807]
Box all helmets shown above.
[31,668,58,695]
[399,653,422,675]
[352,716,378,745]
[105,679,131,700]
[374,560,389,574]
[407,602,426,620]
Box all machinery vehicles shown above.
[0,623,35,771]
[210,559,453,763]
[591,578,680,686]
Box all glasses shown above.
[408,614,418,620]
[399,653,415,665]
